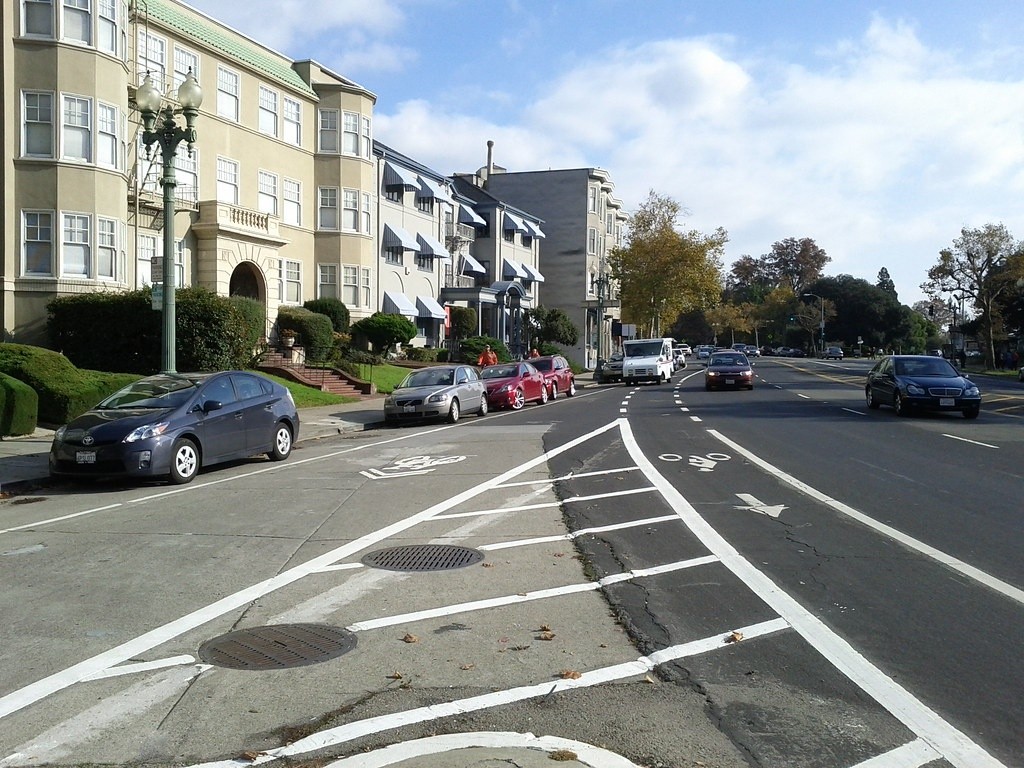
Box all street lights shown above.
[135,66,206,372]
[589,262,613,380]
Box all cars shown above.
[701,351,755,390]
[673,343,843,370]
[603,351,622,379]
[863,354,981,420]
[477,362,549,412]
[48,369,302,484]
[384,364,488,423]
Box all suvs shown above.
[523,355,577,398]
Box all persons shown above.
[477,344,498,368]
[528,348,539,358]
[994,350,1020,370]
[867,348,876,359]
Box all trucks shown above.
[622,339,673,385]
